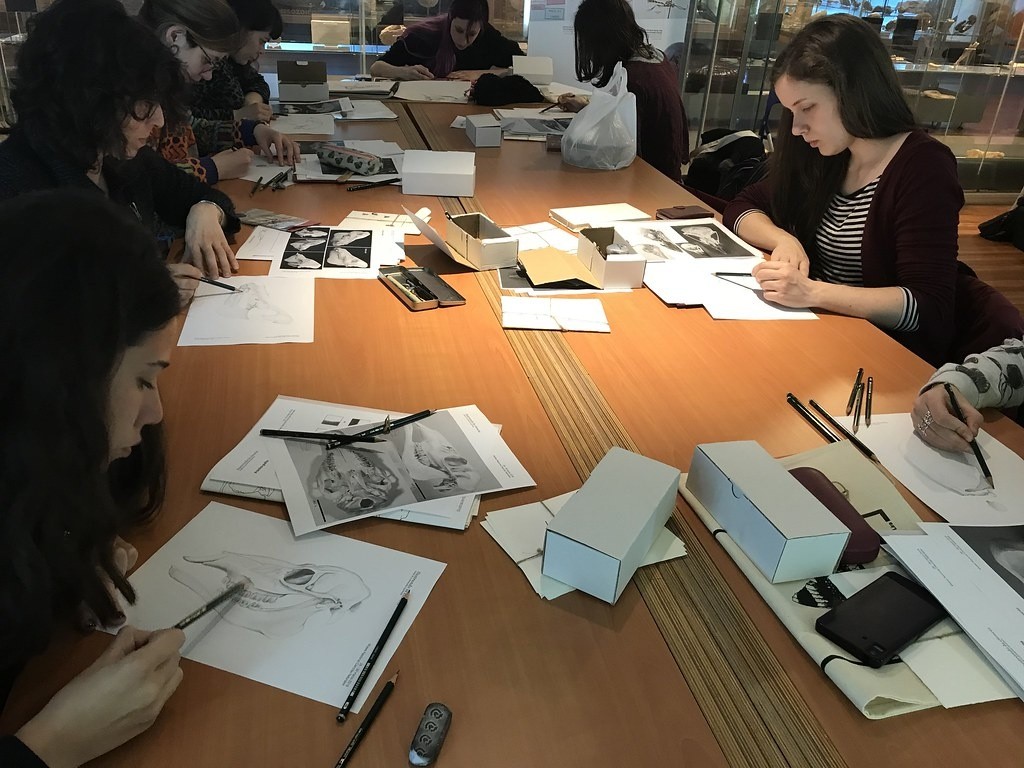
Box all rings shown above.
[922,411,935,426]
[918,420,928,436]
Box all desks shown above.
[399,90,1024,768]
[0,95,784,768]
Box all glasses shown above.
[196,46,226,74]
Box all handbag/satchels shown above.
[561,62,636,170]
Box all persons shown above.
[138,0,302,186]
[370,0,525,81]
[911,334,1024,454]
[0,188,185,768]
[1,0,241,306]
[722,13,965,345]
[558,0,689,181]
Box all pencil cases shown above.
[378,265,466,311]
[789,468,881,565]
[317,144,383,175]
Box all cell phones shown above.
[815,572,947,669]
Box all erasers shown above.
[408,702,452,768]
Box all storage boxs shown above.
[508,56,554,84]
[444,213,519,272]
[542,448,681,604]
[401,150,476,197]
[278,61,330,101]
[576,226,646,287]
[687,438,851,585]
[465,114,501,147]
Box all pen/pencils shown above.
[538,95,575,114]
[943,382,994,489]
[326,408,438,450]
[230,145,237,152]
[181,274,243,292]
[273,113,289,116]
[249,168,294,197]
[336,589,411,723]
[786,368,881,465]
[444,210,454,222]
[334,669,401,768]
[260,429,387,443]
[347,178,401,191]
[712,272,751,276]
[386,274,436,302]
[172,583,244,630]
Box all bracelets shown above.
[199,200,224,224]
